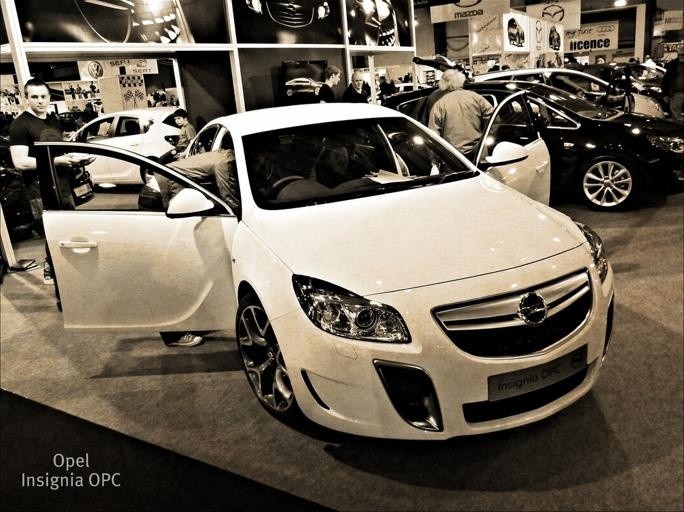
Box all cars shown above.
[0,63,684,440]
[549,25,561,50]
[245,0,337,30]
[508,17,525,47]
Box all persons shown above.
[642,54,657,79]
[340,69,372,103]
[317,63,342,103]
[170,108,197,161]
[661,45,684,123]
[375,57,610,88]
[136,145,239,347]
[624,56,638,81]
[0,94,179,148]
[381,71,400,111]
[8,75,93,284]
[426,68,497,169]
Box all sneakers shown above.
[43,261,54,284]
[165,332,203,347]
